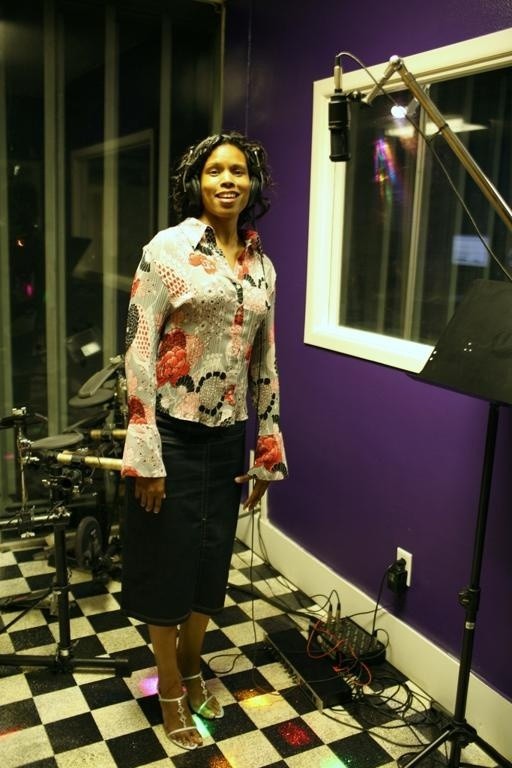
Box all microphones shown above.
[329,57,352,162]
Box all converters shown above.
[384,568,408,595]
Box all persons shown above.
[117,129,291,753]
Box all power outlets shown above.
[395,546,413,588]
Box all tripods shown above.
[1,370,133,678]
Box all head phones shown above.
[183,134,271,214]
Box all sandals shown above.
[179,671,223,718]
[157,682,202,753]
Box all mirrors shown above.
[302,26,511,376]
